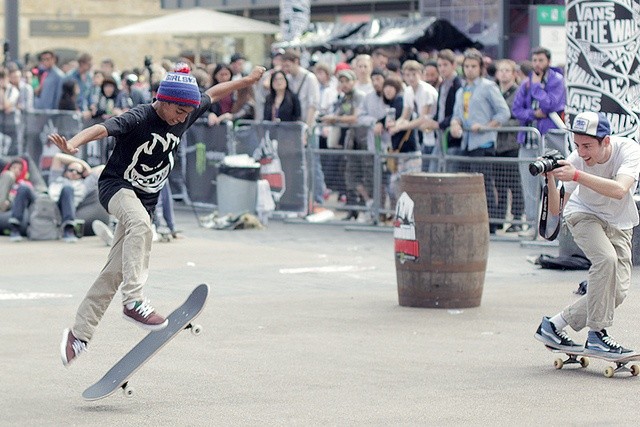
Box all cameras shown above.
[529,148,565,176]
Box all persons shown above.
[48,153,106,231]
[282,53,328,204]
[205,62,255,125]
[265,71,301,121]
[0,158,35,240]
[339,50,568,240]
[230,53,248,80]
[1,51,145,118]
[313,63,338,147]
[533,111,640,360]
[47,65,266,367]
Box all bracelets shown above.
[573,169,579,181]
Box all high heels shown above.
[341,210,359,222]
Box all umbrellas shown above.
[100,7,281,66]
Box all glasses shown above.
[66,168,82,175]
[0,72,6,79]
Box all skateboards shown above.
[543,345,640,377]
[82,284,208,400]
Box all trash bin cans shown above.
[216,165,260,216]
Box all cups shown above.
[385,107,396,123]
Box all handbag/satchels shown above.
[386,147,399,174]
[292,94,301,119]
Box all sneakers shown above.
[490,227,496,234]
[379,213,386,225]
[61,224,78,243]
[323,189,331,199]
[121,296,169,331]
[497,225,504,229]
[93,220,115,247]
[151,224,162,242]
[518,225,536,237]
[9,225,20,241]
[506,224,523,232]
[583,328,637,359]
[60,327,88,369]
[386,214,396,225]
[361,213,375,225]
[365,199,374,208]
[533,314,585,352]
[340,195,346,204]
[535,234,545,241]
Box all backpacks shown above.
[26,194,63,240]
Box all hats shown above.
[100,74,116,85]
[370,67,389,80]
[564,112,611,139]
[271,48,286,59]
[156,62,201,110]
[335,62,352,74]
[315,62,334,78]
[337,69,357,80]
[228,52,248,66]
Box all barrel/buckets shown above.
[391,171,493,310]
